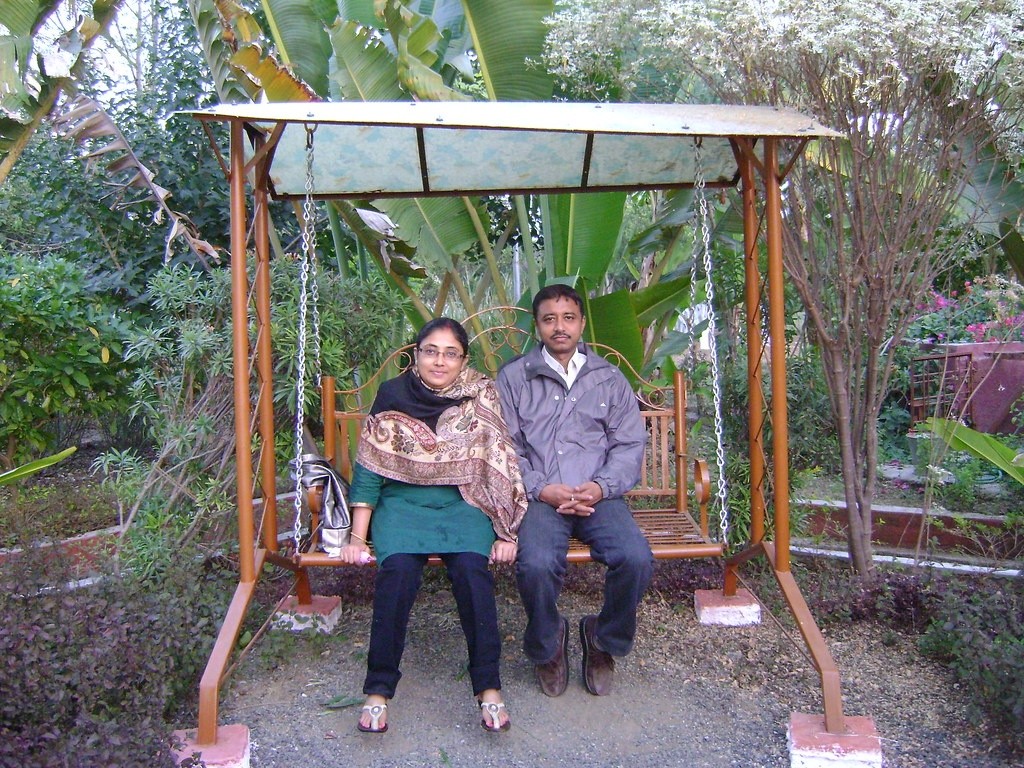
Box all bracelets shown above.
[350,532,367,542]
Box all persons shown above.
[340,318,518,734]
[498,284,655,697]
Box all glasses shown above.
[417,345,465,359]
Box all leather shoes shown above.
[533,616,570,697]
[579,613,616,695]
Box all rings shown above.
[570,494,574,501]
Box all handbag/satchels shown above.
[290,454,352,554]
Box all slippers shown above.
[478,697,511,734]
[358,703,390,733]
